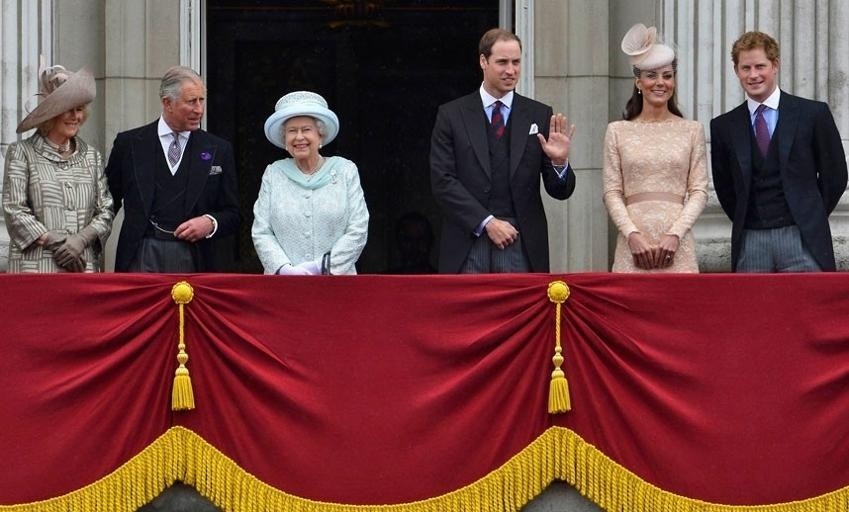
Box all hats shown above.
[16,64,97,134]
[264,91,340,150]
[621,23,675,71]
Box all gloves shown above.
[278,264,313,275]
[43,228,87,272]
[294,260,321,276]
[54,225,98,268]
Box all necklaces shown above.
[43,134,71,152]
[296,155,321,175]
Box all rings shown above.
[666,255,672,262]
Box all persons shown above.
[429,27,577,273]
[707,32,849,276]
[602,46,709,274]
[94,65,241,275]
[251,91,369,277]
[0,63,116,273]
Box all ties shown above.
[168,132,181,168]
[754,105,770,160]
[491,101,505,140]
